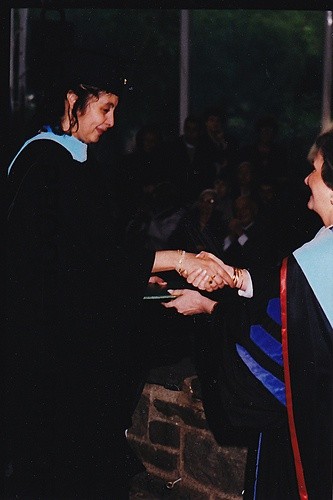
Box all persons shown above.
[121,109,307,261]
[160,132,333,500]
[1,58,231,500]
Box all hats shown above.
[61,52,127,98]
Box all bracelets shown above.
[232,268,244,290]
[173,250,186,274]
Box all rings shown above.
[212,275,216,279]
[209,281,213,285]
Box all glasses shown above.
[199,197,215,205]
